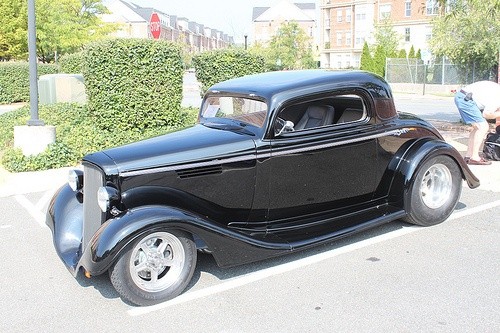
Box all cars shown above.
[45,69,480,306]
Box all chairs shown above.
[337,108,363,123]
[294,103,334,130]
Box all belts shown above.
[460,90,472,100]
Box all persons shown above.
[454,81,500,165]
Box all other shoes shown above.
[469,158,492,164]
[465,157,470,163]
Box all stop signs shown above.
[149,11,161,39]
